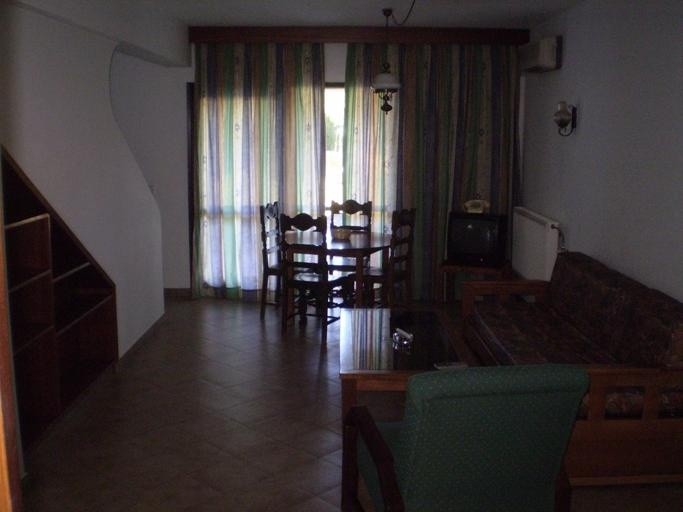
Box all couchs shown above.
[335,244,681,510]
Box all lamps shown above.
[552,98,577,138]
[367,6,402,114]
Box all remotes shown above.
[434,361,469,370]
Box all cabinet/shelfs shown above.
[3,205,63,459]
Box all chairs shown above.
[334,358,593,511]
[360,205,415,310]
[258,199,325,323]
[325,196,375,307]
[278,207,353,350]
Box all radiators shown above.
[508,204,560,285]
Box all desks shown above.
[438,260,505,303]
[273,230,400,326]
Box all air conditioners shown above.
[511,32,560,77]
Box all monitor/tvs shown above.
[447,211,509,267]
